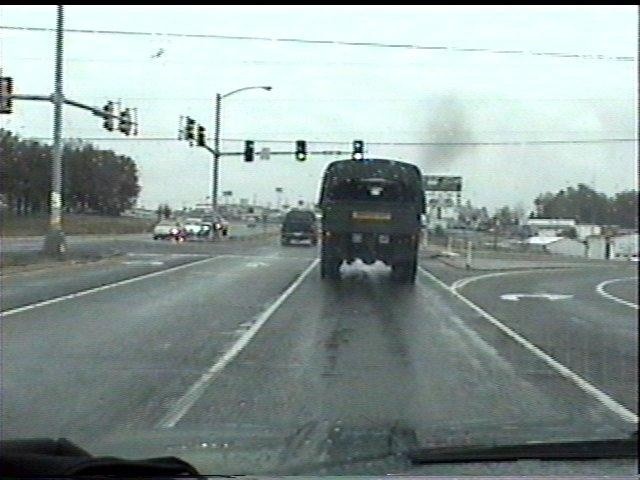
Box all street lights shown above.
[208,83,273,216]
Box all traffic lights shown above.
[121,107,129,133]
[184,116,197,141]
[295,140,308,162]
[243,140,254,162]
[196,124,206,147]
[351,140,364,161]
[103,101,110,130]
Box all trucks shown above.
[317,158,426,286]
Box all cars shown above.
[151,210,228,242]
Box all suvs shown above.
[280,209,320,247]
